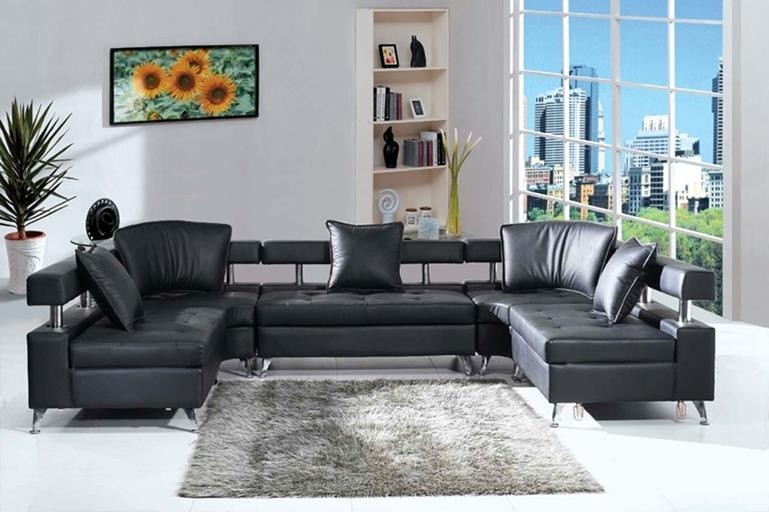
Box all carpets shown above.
[178,378,606,498]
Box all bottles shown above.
[405,207,432,228]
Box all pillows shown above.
[75,246,146,331]
[500,220,618,301]
[112,220,232,297]
[590,238,659,324]
[325,219,406,294]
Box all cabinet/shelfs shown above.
[355,7,450,226]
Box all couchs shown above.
[26,238,715,435]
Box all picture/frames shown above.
[378,44,400,68]
[408,98,427,118]
[109,43,259,126]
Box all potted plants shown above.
[0,95,80,297]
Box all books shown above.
[402,130,447,167]
[373,83,403,121]
